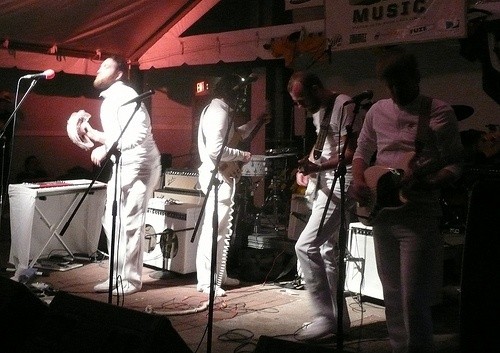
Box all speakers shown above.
[344,222,384,305]
[34,291,193,352]
[0,276,45,352]
[142,198,207,276]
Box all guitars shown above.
[219,110,268,183]
[357,150,461,226]
[297,156,319,195]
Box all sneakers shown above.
[112,279,143,296]
[94,278,120,291]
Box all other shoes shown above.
[223,277,239,285]
[279,274,304,289]
[302,322,352,337]
[295,318,332,341]
[196,284,226,297]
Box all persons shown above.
[352,59,462,352]
[196,75,252,297]
[81,56,162,297]
[287,70,370,338]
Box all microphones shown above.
[21,69,55,80]
[291,211,310,219]
[341,89,374,107]
[344,258,364,262]
[231,72,258,92]
[122,89,156,106]
